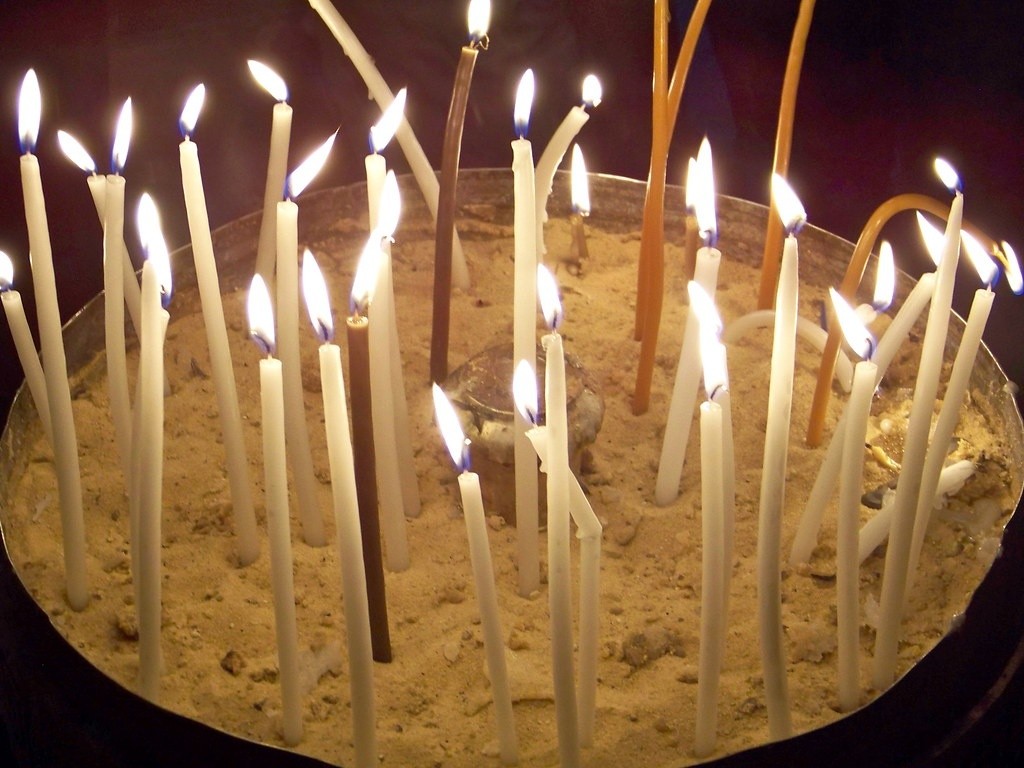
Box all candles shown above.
[0,1,1024,768]
